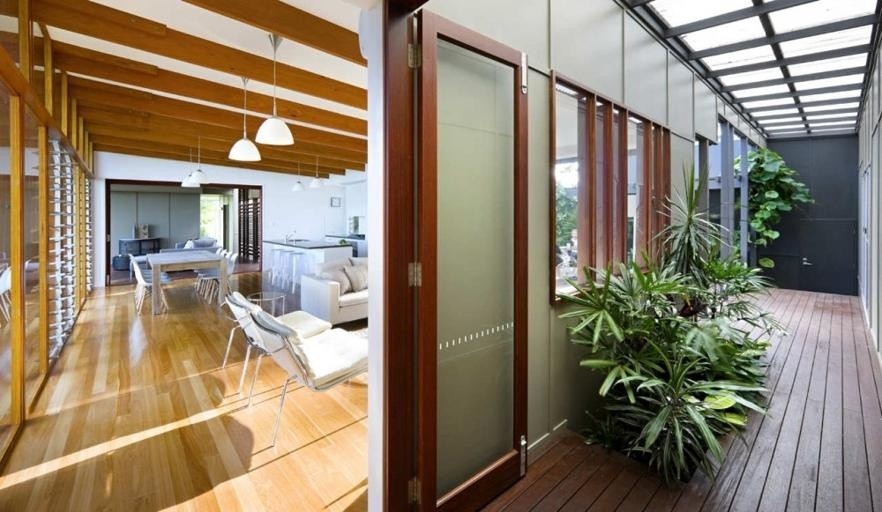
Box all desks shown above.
[246,291,287,316]
[119,238,160,256]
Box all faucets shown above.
[285,229,297,245]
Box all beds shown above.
[128,238,219,280]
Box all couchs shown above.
[300,272,369,325]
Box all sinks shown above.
[289,239,310,243]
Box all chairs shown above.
[222,291,333,394]
[246,310,368,447]
[128,247,239,315]
[0,257,32,329]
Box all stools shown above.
[267,248,306,294]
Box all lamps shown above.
[255,34,294,146]
[181,144,201,188]
[228,76,262,161]
[293,161,305,191]
[188,135,210,184]
[309,155,325,188]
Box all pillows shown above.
[311,256,368,296]
[184,238,216,249]
